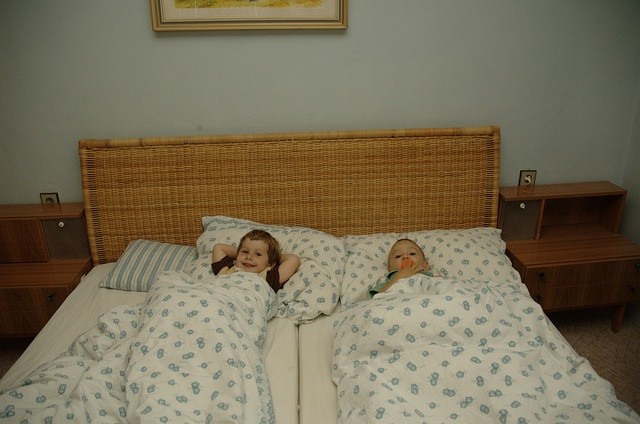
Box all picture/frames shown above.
[518,170,537,188]
[150,0,348,32]
[40,192,62,213]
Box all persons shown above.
[368,236,434,298]
[212,228,301,293]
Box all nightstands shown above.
[497,181,640,333]
[0,203,94,337]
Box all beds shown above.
[1,126,640,424]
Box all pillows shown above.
[339,227,521,312]
[196,215,350,324]
[98,239,198,291]
